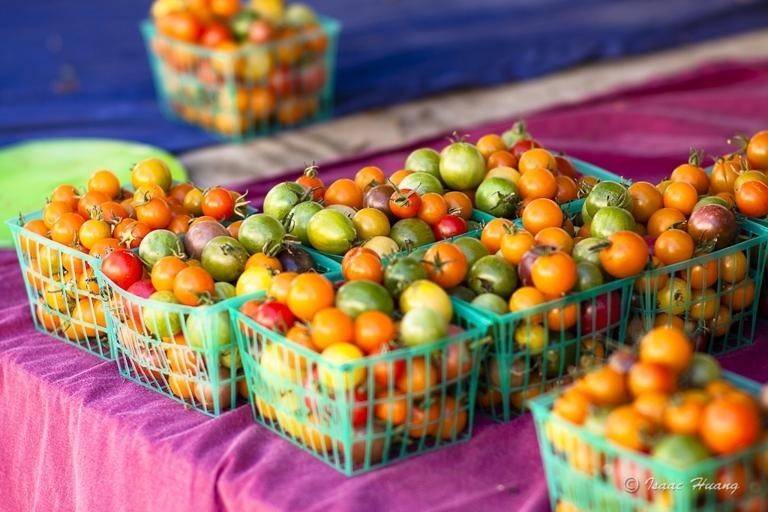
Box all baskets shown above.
[140,18,342,143]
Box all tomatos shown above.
[15,0,768,511]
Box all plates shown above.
[0,137,187,251]
